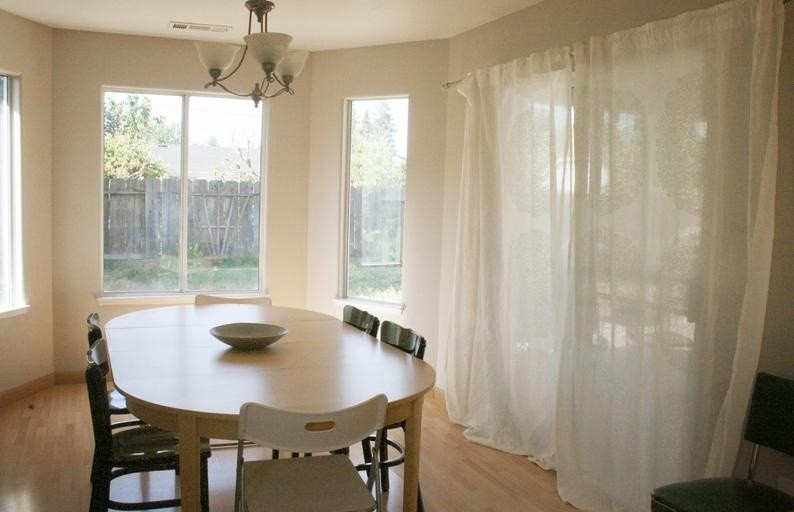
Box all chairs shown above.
[234,395,388,512]
[269,306,406,459]
[652,373,794,512]
[195,295,273,307]
[86,314,210,512]
[352,321,426,512]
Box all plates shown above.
[209,323,288,350]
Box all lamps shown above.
[193,0,311,109]
